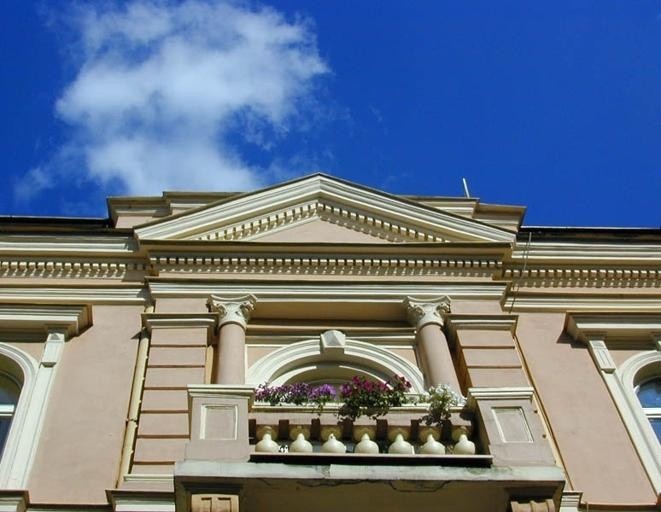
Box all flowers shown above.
[253,374,470,427]
